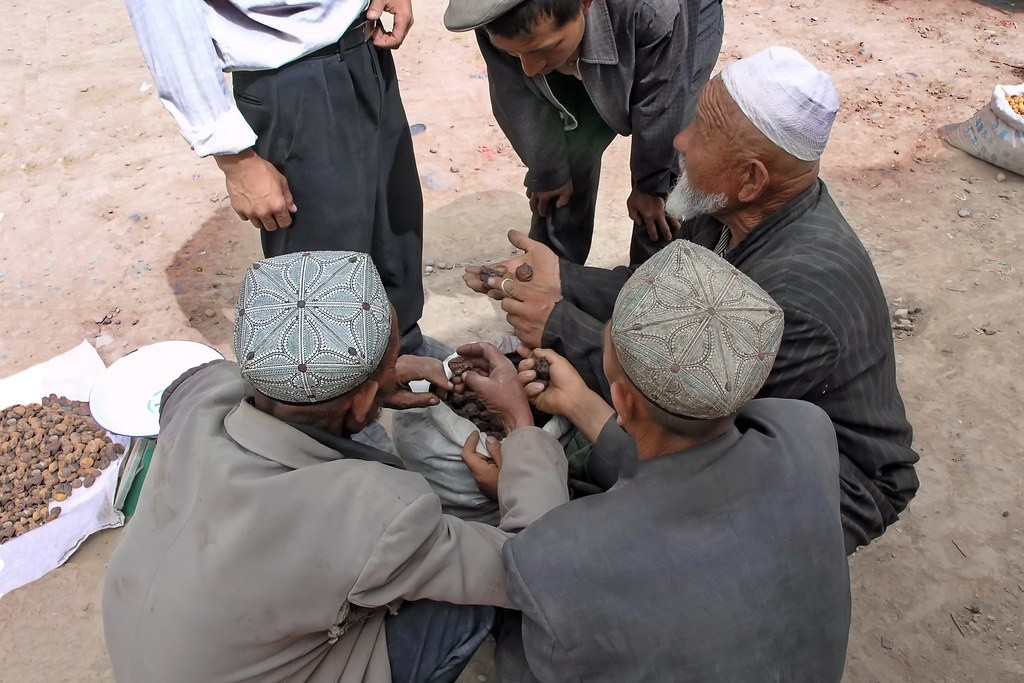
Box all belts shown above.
[301,18,379,58]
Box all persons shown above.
[462,43,920,545]
[459,242,853,683]
[128,0,458,362]
[443,0,726,266]
[103,251,570,683]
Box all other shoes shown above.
[411,336,455,361]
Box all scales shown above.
[88,340,225,516]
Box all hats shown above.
[721,46,838,159]
[444,0,520,32]
[612,240,784,420]
[232,249,392,404]
